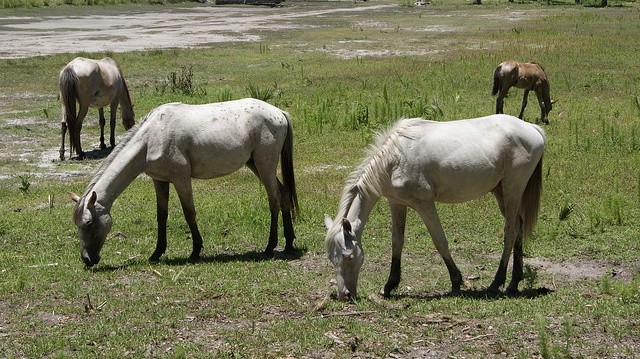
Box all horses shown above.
[58,57,135,161]
[65,97,300,268]
[491,59,558,125]
[323,114,547,301]
